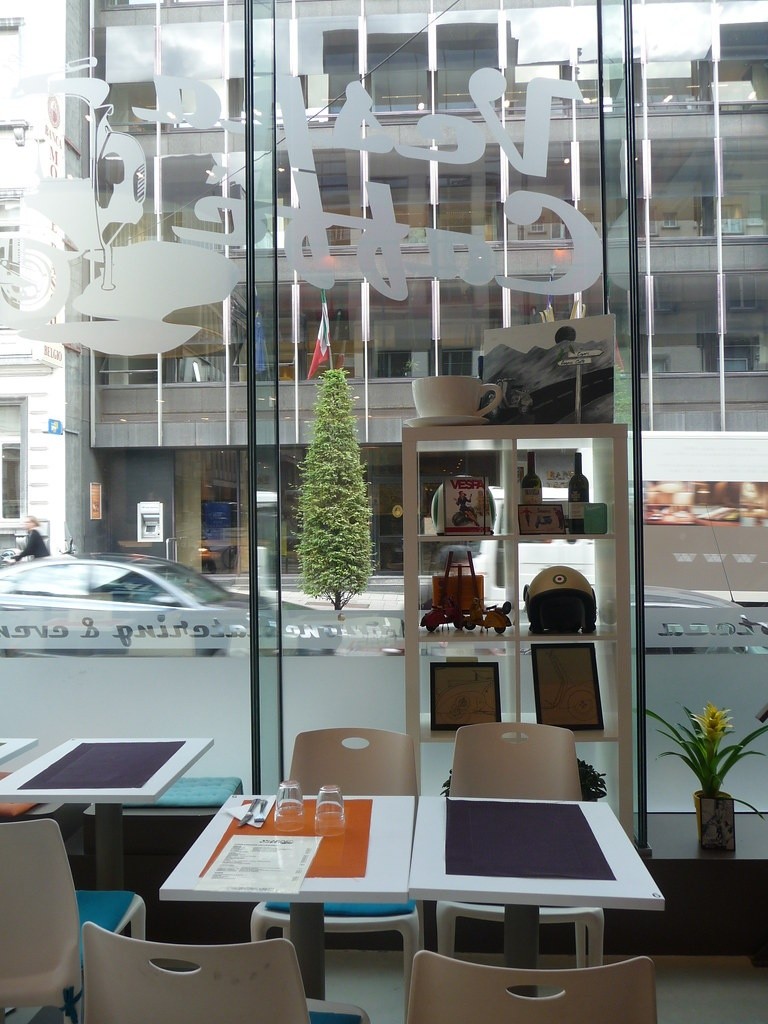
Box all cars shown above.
[522,585,745,611]
[0,552,345,657]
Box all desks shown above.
[159,791,668,999]
[0,740,215,893]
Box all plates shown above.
[403,416,490,426]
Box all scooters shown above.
[192,529,218,572]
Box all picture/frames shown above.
[699,798,736,852]
[531,643,605,733]
[429,661,501,732]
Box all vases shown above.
[693,785,732,832]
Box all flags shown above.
[306,289,331,381]
[255,295,269,374]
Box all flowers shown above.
[646,702,768,821]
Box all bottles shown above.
[520,452,542,505]
[568,452,589,534]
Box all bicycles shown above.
[221,547,237,569]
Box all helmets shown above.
[523,567,599,634]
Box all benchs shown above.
[123,773,244,818]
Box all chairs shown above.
[81,921,370,1023]
[0,817,149,1024]
[249,726,424,1024]
[406,949,659,1024]
[434,723,605,968]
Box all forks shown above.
[254,799,268,822]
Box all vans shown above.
[477,486,597,620]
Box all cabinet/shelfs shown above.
[401,423,629,854]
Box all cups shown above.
[273,781,306,831]
[315,785,345,837]
[412,375,501,417]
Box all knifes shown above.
[237,799,260,828]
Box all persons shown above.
[11,515,50,561]
